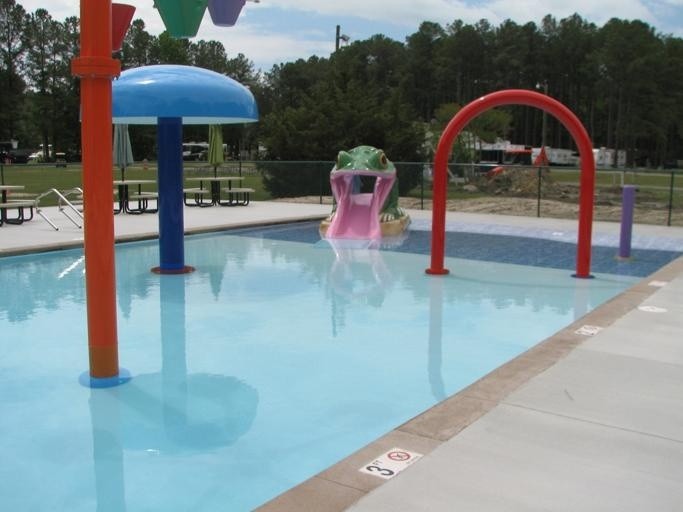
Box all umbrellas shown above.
[112,124,134,181]
[207,124,224,178]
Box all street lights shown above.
[535,81,548,218]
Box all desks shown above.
[113,179,156,213]
[0,186,25,221]
[186,177,244,206]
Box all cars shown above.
[181,143,229,162]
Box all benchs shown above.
[0,202,34,227]
[6,199,40,221]
[183,188,255,207]
[114,189,158,215]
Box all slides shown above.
[325,177,397,242]
